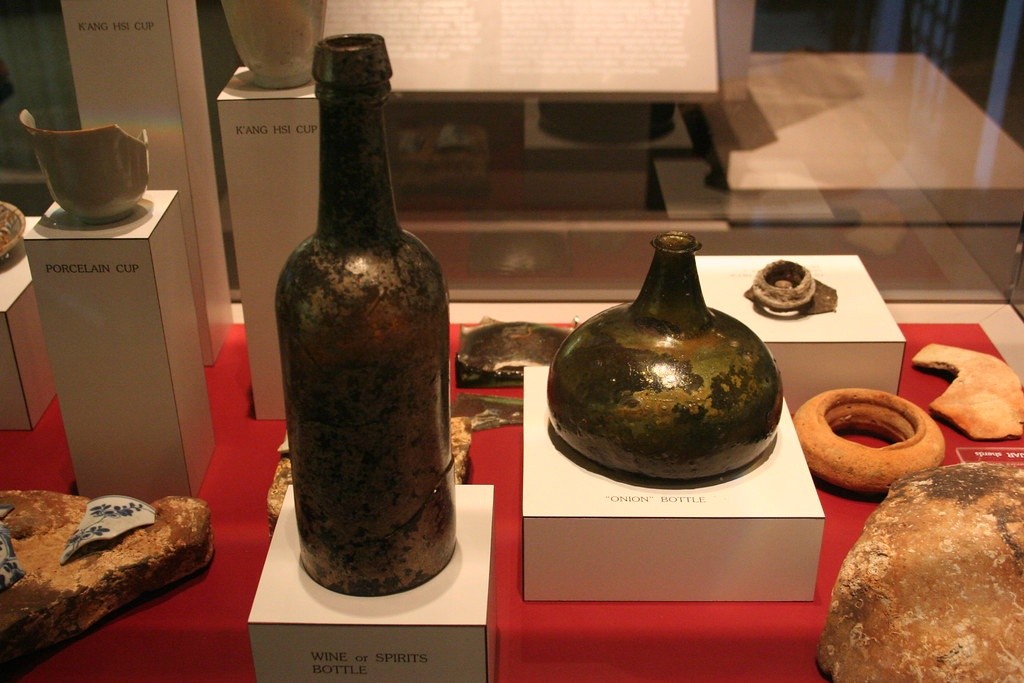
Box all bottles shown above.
[275,33,456,598]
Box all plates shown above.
[0,201,26,262]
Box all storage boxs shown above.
[0,0,908,682]
